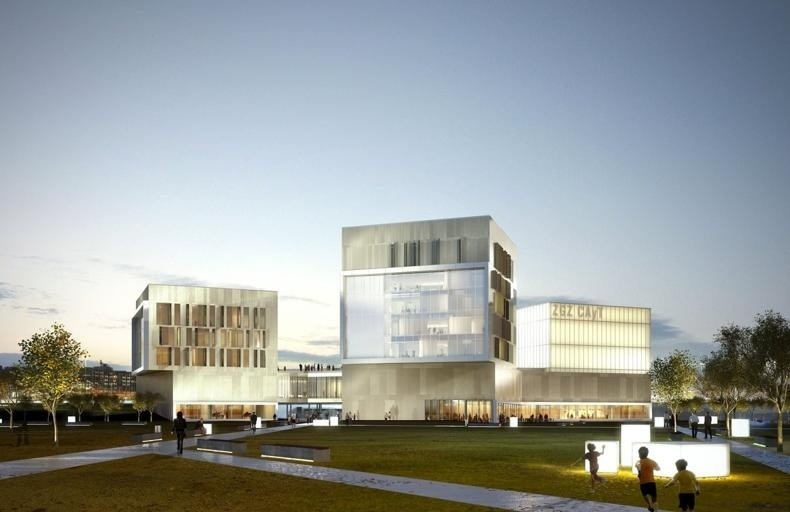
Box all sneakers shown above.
[587,476,659,512]
[664,425,713,440]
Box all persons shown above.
[277,362,335,372]
[570,442,608,490]
[663,411,679,429]
[216,405,392,435]
[635,446,661,512]
[664,459,702,512]
[703,410,713,439]
[390,280,449,358]
[172,411,188,455]
[688,410,700,438]
[196,416,207,435]
[421,409,610,427]
